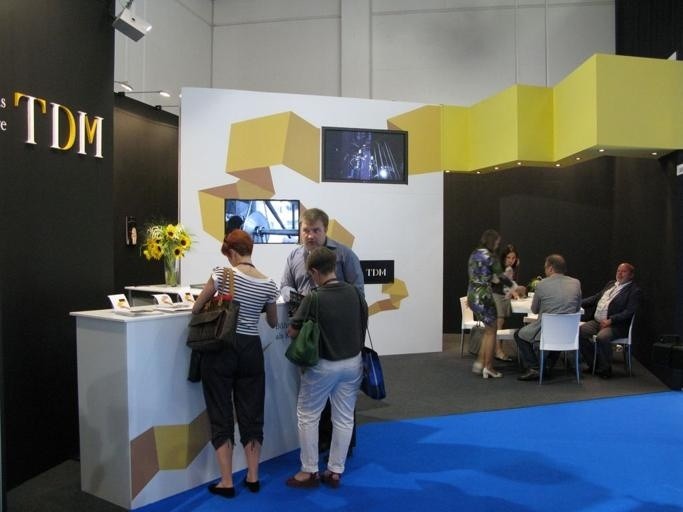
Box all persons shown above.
[280,207,365,462]
[466,228,526,380]
[284,245,370,489]
[513,254,582,382]
[192,229,279,499]
[492,244,520,362]
[581,263,636,379]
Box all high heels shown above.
[472,361,483,374]
[483,367,503,379]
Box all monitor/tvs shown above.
[321,125,409,184]
[224,198,301,245]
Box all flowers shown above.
[140,222,197,285]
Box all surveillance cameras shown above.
[111,7,153,41]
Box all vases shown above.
[163,254,182,285]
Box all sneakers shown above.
[208,482,235,498]
[244,475,260,493]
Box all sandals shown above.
[285,472,320,487]
[495,349,512,361]
[320,471,341,488]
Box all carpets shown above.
[128,391,682,511]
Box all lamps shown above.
[114,81,132,91]
[119,90,171,99]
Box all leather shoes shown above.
[517,366,540,380]
[323,449,353,464]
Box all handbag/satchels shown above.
[186,267,240,351]
[355,286,386,400]
[285,290,321,367]
[468,318,485,355]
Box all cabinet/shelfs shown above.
[69,284,302,511]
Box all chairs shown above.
[541,312,580,386]
[460,297,486,356]
[496,328,519,364]
[590,312,635,374]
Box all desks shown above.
[509,288,538,313]
